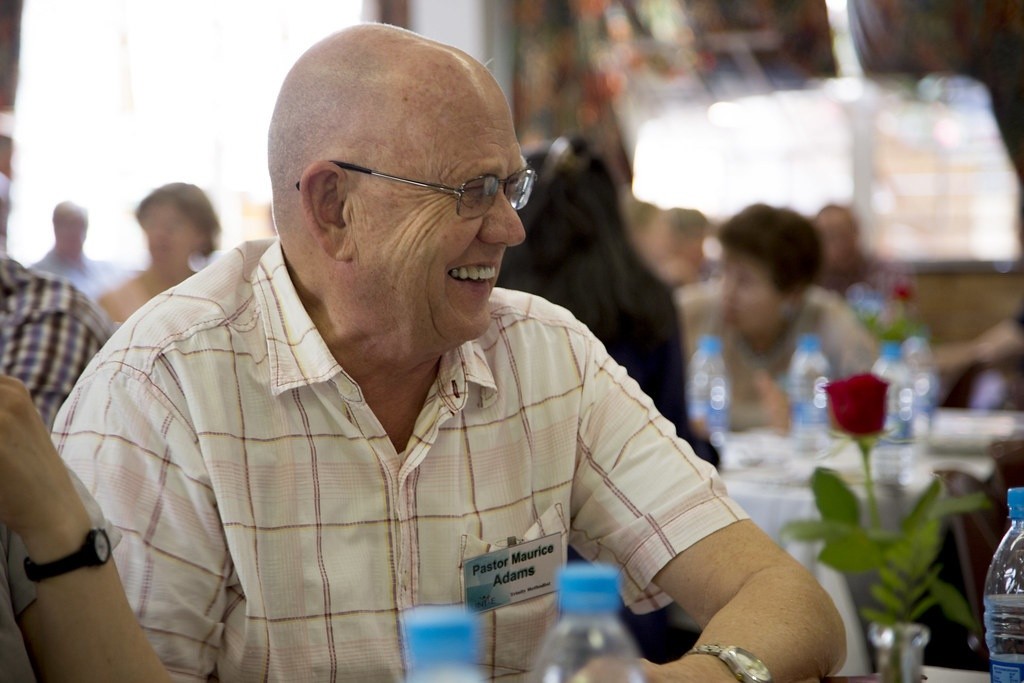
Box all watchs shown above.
[683,639,771,683]
[21,524,110,586]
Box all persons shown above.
[449,130,720,650]
[58,18,854,679]
[92,178,228,332]
[24,196,126,326]
[0,314,177,682]
[607,161,1024,658]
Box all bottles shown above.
[403,608,484,683]
[872,342,915,482]
[687,337,729,450]
[789,334,830,454]
[981,486,1024,683]
[903,332,936,455]
[528,565,640,681]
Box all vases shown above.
[866,623,929,683]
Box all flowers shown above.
[863,278,920,341]
[785,371,990,622]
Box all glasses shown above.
[297,161,539,219]
